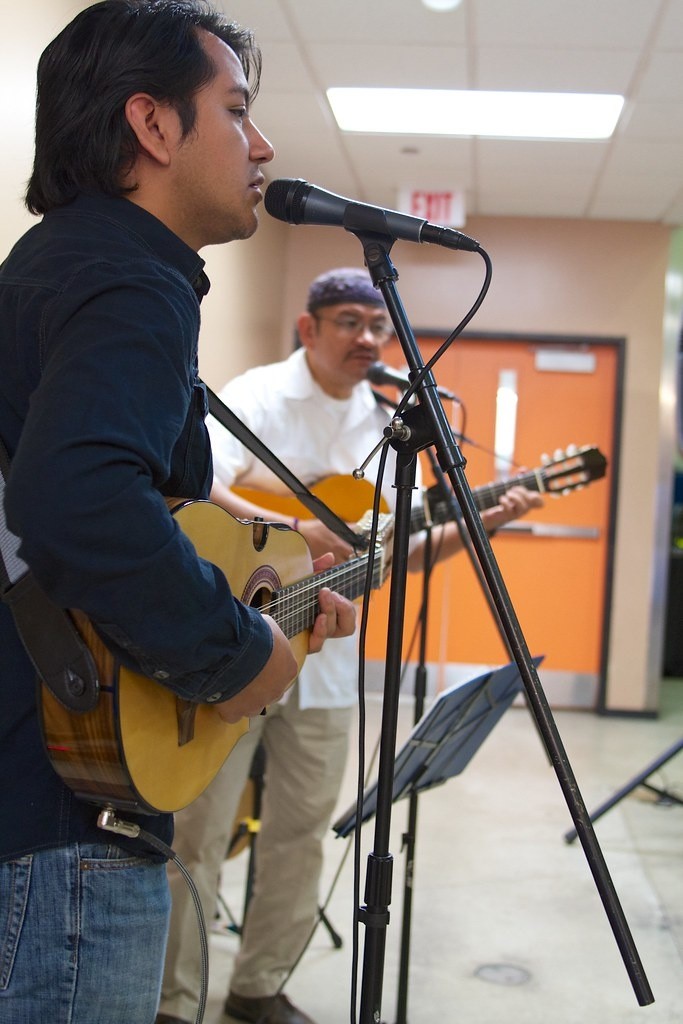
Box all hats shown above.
[307,267,388,311]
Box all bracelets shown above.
[292,517,300,531]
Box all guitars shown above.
[220,443,608,537]
[36,495,395,819]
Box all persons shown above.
[155,265,545,1024]
[0,0,359,1024]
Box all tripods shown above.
[225,746,344,948]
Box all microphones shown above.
[367,362,457,400]
[265,178,481,254]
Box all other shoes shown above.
[225,991,315,1024]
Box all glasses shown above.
[314,314,395,339]
